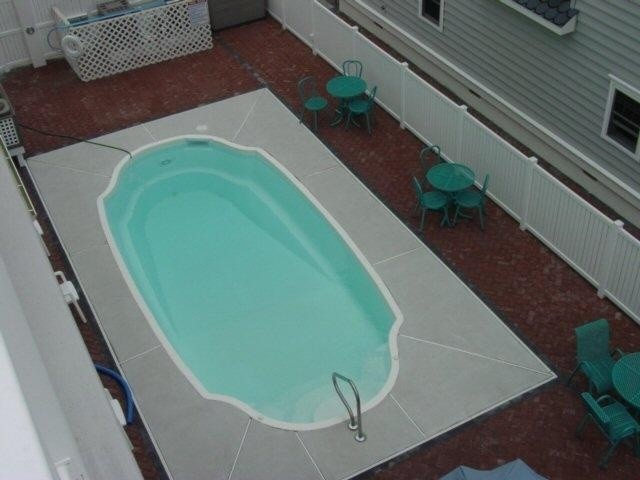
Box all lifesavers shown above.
[61,34,83,58]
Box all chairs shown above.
[298,57,378,138]
[570,318,639,466]
[413,145,490,236]
